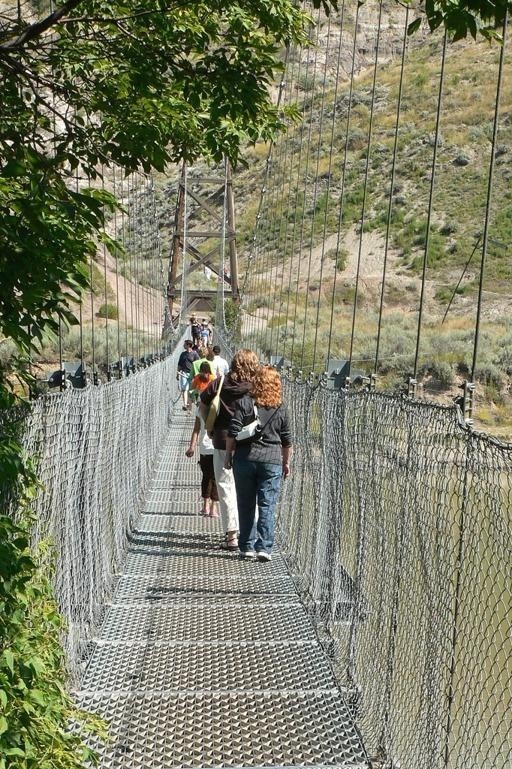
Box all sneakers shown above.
[239,550,255,558]
[257,551,272,562]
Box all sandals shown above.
[221,538,239,550]
[211,511,220,517]
[200,509,209,516]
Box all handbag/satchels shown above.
[235,397,264,445]
[190,390,199,404]
[204,375,225,432]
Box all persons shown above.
[183,380,230,518]
[219,363,298,563]
[177,314,232,418]
[197,347,268,551]
